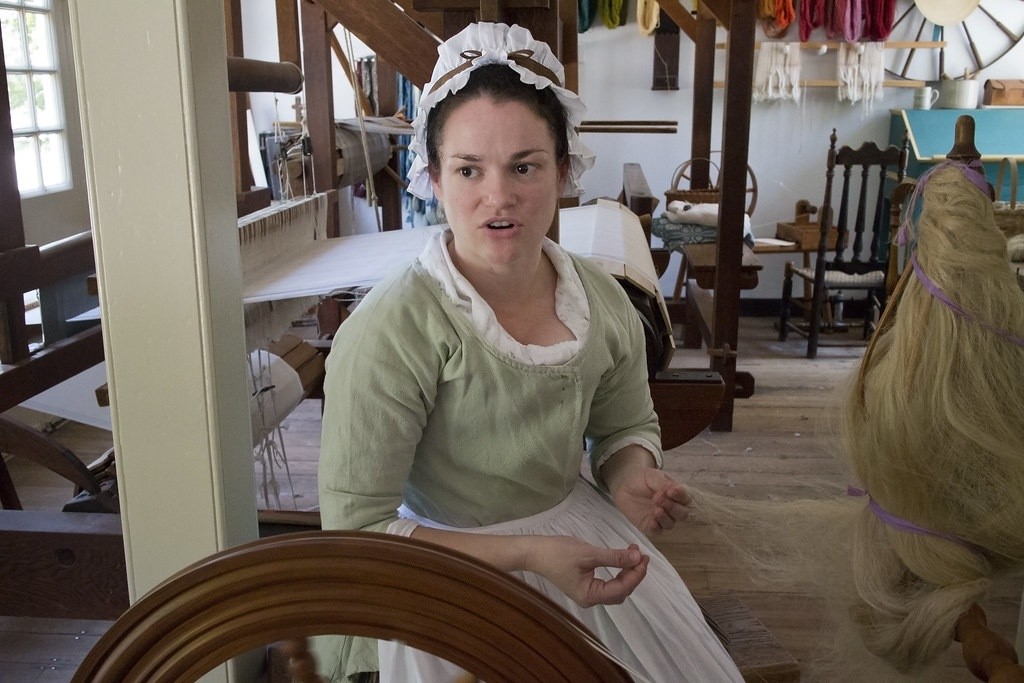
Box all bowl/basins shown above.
[938,80,979,110]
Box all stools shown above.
[695,594,800,683]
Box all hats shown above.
[405,21,599,202]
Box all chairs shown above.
[779,128,911,360]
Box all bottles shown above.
[834,289,844,321]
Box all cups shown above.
[913,86,939,110]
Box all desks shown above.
[752,238,834,328]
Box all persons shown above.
[317,23,746,683]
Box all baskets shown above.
[990,157,1023,234]
[664,158,722,213]
